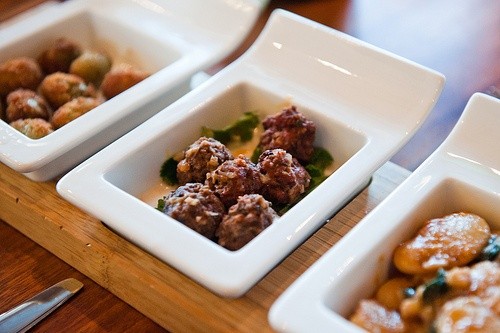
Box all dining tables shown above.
[0,1,499,333]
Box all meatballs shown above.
[165,103,317,251]
[1,37,151,138]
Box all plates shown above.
[55,8,447,300]
[0,0,269,183]
[266,92,500,333]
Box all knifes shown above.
[0,277,84,333]
